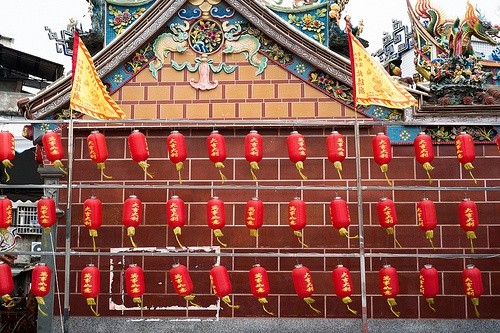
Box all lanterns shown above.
[0,262,482,317]
[0,194,479,254]
[0,130,500,185]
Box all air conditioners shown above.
[31,242,41,257]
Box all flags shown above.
[70,29,126,120]
[348,25,418,109]
